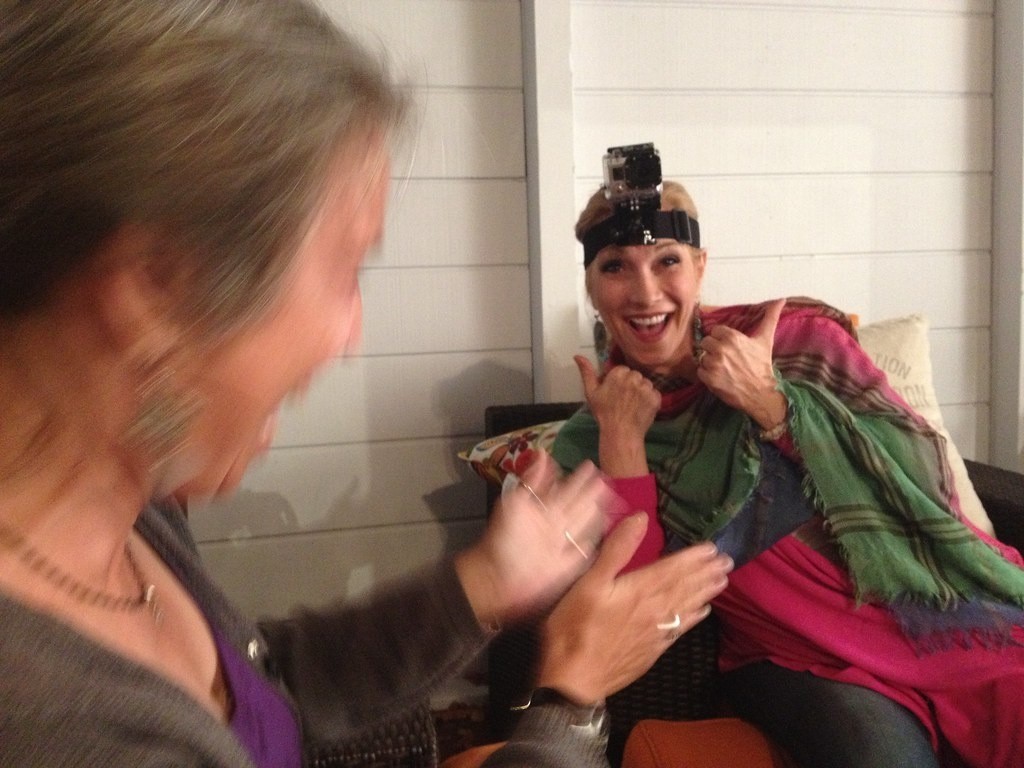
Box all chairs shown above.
[481,312,1024,768]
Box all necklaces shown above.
[2,532,167,625]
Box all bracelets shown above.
[759,421,787,442]
[508,685,571,709]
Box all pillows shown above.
[456,314,999,635]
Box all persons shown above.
[1,0,733,767]
[553,178,1024,768]
